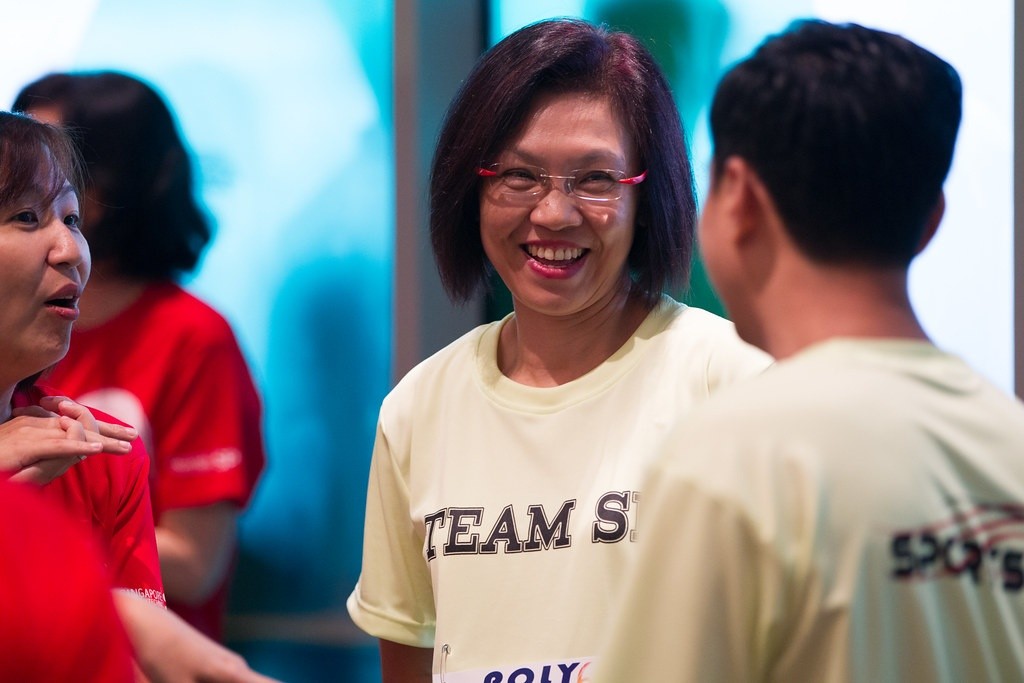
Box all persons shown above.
[345,18,777,683]
[594,22,1022,683]
[0,71,287,683]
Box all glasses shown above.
[472,164,647,202]
